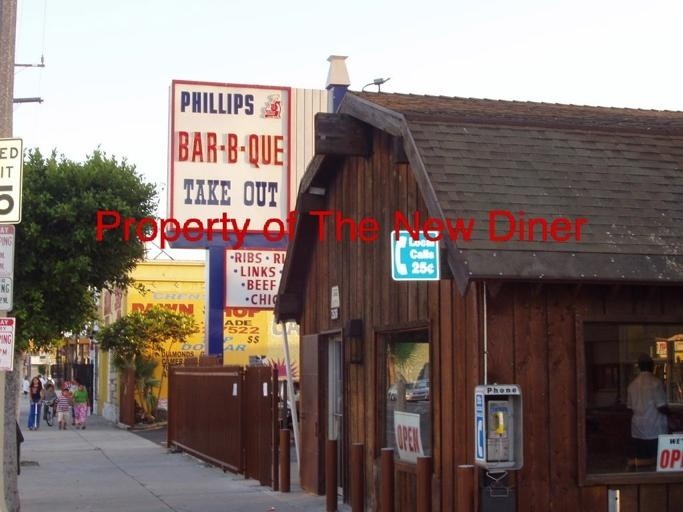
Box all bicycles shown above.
[41,396,58,427]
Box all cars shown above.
[386,380,429,403]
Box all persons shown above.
[72,383,90,429]
[21,374,81,395]
[26,377,45,431]
[54,388,73,430]
[41,384,57,420]
[625,351,683,460]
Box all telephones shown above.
[488,401,513,461]
